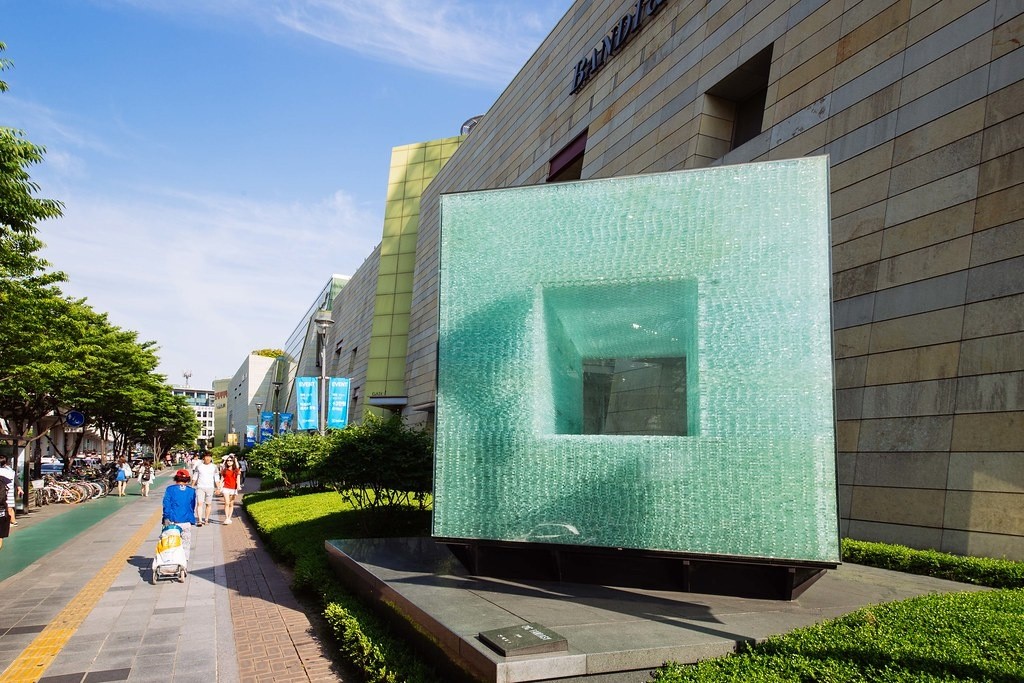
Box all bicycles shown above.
[29,447,153,508]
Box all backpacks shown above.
[142,465,151,481]
[116,470,127,481]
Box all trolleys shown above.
[151,523,191,585]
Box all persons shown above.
[162,470,197,568]
[0,450,250,550]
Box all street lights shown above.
[313,310,335,438]
[255,403,264,443]
[272,381,284,433]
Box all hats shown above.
[176,470,188,478]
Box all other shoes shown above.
[223,518,232,525]
[10,522,18,526]
[205,520,209,525]
[197,521,202,526]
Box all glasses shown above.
[227,460,232,462]
